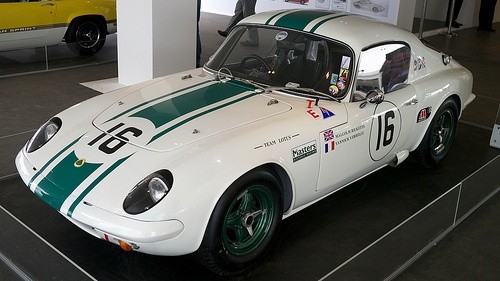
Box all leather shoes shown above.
[240,40,259,47]
[477,26,496,32]
[217,30,228,37]
[445,19,463,27]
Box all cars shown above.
[1,1,118,53]
[14,9,477,260]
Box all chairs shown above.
[313,52,352,97]
[264,39,306,86]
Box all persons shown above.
[218,0,260,48]
[446,0,463,29]
[197,1,203,68]
[478,0,497,32]
